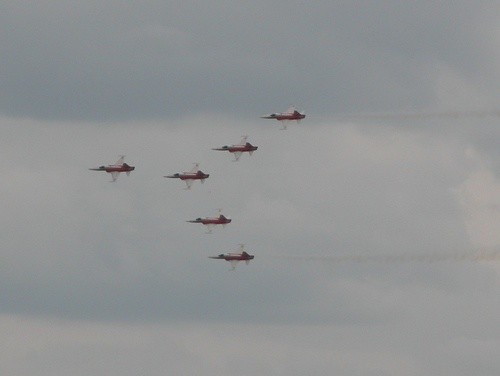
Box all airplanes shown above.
[90,107,305,263]
